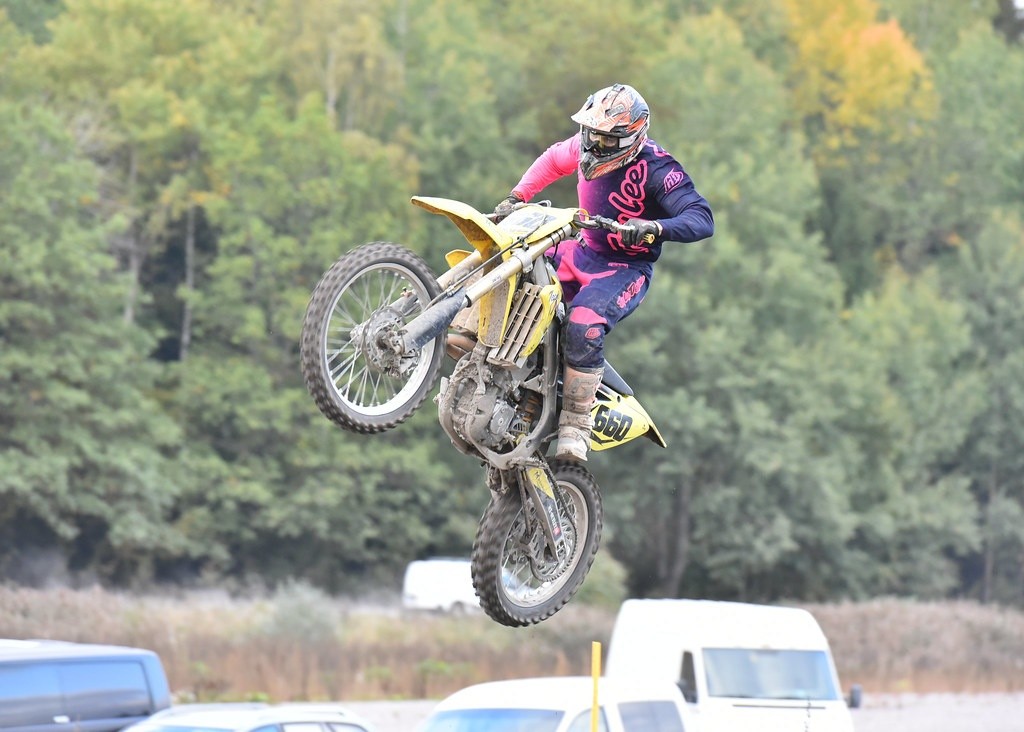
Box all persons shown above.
[494,82,715,463]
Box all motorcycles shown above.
[300,196,667,627]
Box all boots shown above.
[446,332,478,362]
[555,365,605,463]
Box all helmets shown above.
[570,83,651,180]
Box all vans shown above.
[402,560,536,616]
[0,640,173,732]
[604,598,862,732]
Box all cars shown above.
[409,678,694,732]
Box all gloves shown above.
[622,219,660,250]
[495,194,523,225]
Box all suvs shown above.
[120,705,372,732]
